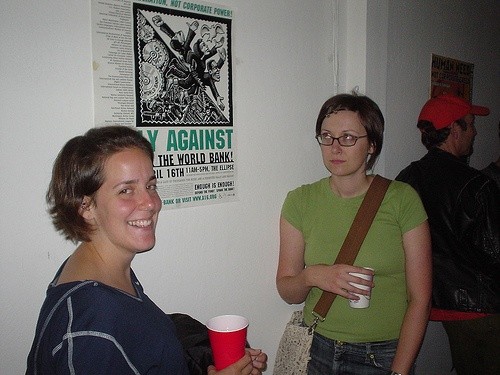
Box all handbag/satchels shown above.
[273,310,314,375]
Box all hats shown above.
[417,94,491,133]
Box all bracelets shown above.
[388,371,402,375]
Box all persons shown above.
[25,125,268,375]
[276,93,433,375]
[395,91,500,375]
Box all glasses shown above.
[315,134,369,146]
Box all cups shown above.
[348,266,374,309]
[206,315,249,370]
